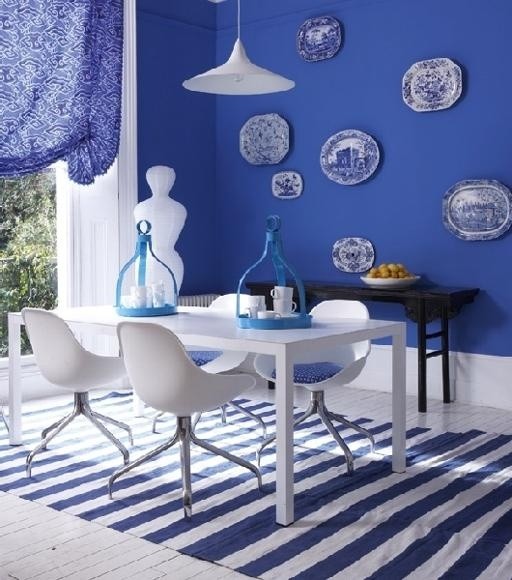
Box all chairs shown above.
[108,321,262,520]
[134,293,267,445]
[20,307,134,477]
[253,300,375,475]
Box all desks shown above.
[245,278,481,413]
[7,306,406,528]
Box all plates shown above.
[331,237,374,275]
[401,58,463,113]
[441,179,512,241]
[318,129,380,186]
[295,16,342,63]
[359,276,421,290]
[271,171,303,200]
[239,113,290,167]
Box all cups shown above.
[248,296,265,306]
[246,306,266,319]
[270,286,293,299]
[272,299,297,315]
[141,287,152,308]
[152,284,166,307]
[257,311,281,320]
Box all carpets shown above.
[1,389,512,579]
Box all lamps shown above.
[182,0,295,96]
